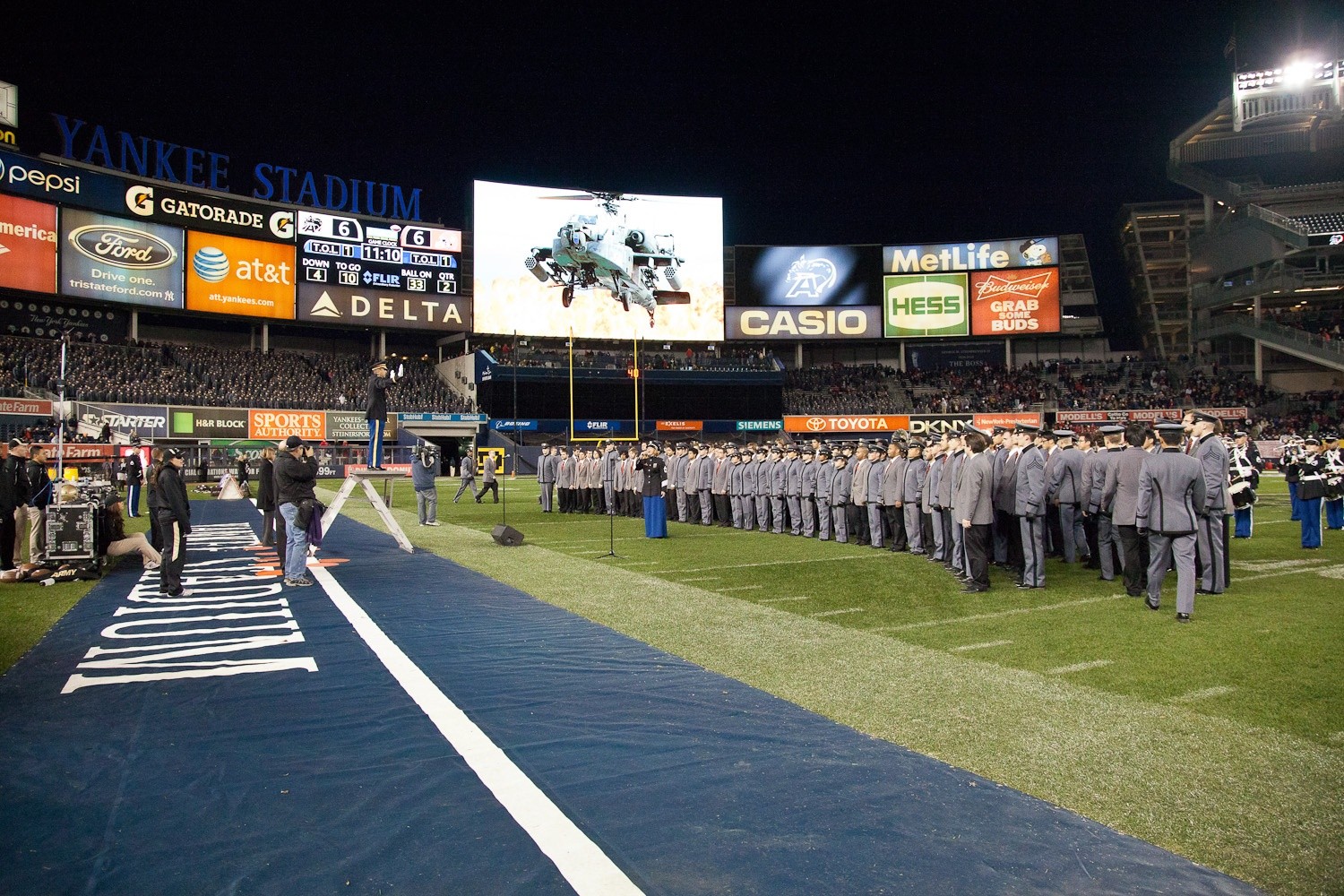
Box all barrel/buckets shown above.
[48,467,57,480]
[64,467,78,482]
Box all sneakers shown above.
[143,560,161,570]
[283,578,314,587]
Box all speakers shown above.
[491,524,524,546]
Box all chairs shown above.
[1283,212,1344,234]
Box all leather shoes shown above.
[854,539,1231,623]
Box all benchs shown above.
[776,361,1246,413]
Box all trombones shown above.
[1273,439,1305,452]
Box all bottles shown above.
[84,478,89,485]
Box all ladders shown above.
[307,475,413,554]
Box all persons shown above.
[0,327,485,416]
[155,448,193,598]
[410,446,440,526]
[453,447,478,504]
[1136,424,1207,623]
[536,408,1344,596]
[486,341,774,371]
[476,450,499,504]
[784,298,1344,442]
[319,437,456,478]
[365,360,403,470]
[0,416,318,587]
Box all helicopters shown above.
[522,189,691,316]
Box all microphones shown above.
[501,454,511,459]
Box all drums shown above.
[1323,476,1344,501]
[1228,481,1259,510]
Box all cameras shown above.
[302,445,310,457]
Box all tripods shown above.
[596,459,625,560]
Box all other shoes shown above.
[13,560,23,568]
[419,521,439,526]
[31,560,45,565]
[159,588,193,597]
[368,466,386,470]
[133,514,143,517]
[475,496,481,503]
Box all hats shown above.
[369,360,388,370]
[539,410,1343,459]
[9,438,29,449]
[164,448,185,458]
[286,436,308,449]
[132,442,142,447]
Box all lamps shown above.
[1018,238,1045,255]
